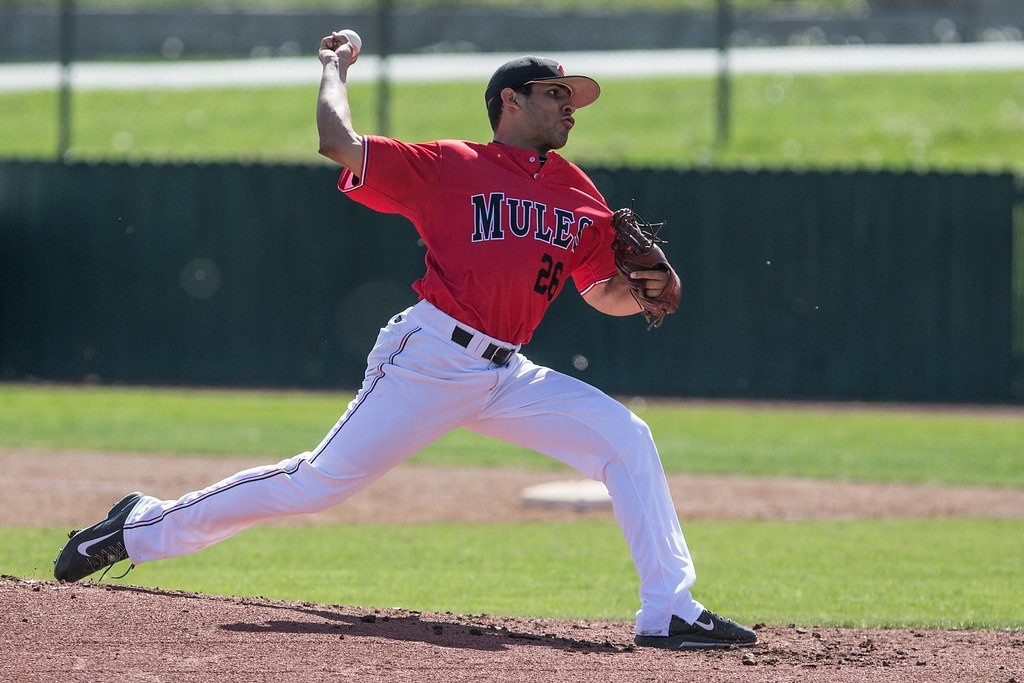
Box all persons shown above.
[54,28,760,650]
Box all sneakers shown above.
[52,492,146,583]
[634,606,758,646]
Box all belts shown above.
[452,326,515,365]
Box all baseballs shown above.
[334,29,363,58]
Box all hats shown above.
[484,56,601,109]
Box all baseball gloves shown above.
[609,207,683,318]
